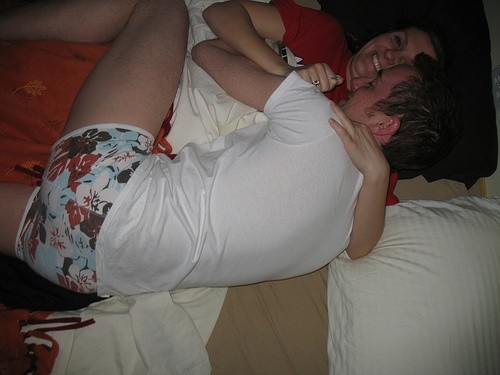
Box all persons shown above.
[0,0,447,262]
[0,0,463,298]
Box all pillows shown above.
[324,195,498,374]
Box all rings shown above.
[328,75,340,81]
[311,80,320,86]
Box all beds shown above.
[4,7,496,373]
